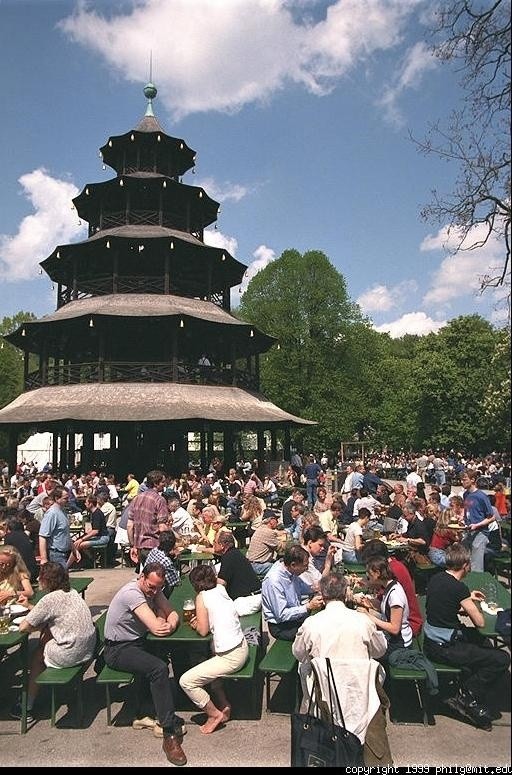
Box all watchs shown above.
[13,590,16,598]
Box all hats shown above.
[204,473,215,481]
[209,515,227,525]
[262,511,281,519]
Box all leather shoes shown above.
[162,734,187,766]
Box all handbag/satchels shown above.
[290,713,364,768]
[495,609,512,636]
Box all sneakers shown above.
[9,708,34,723]
[448,688,502,729]
[133,717,186,737]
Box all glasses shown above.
[142,582,166,593]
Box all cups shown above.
[0,607,11,636]
[183,599,196,625]
[374,528,380,540]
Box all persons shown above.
[356,539,423,638]
[261,545,326,641]
[422,548,510,723]
[0,448,512,595]
[290,573,393,768]
[213,531,262,616]
[0,545,35,604]
[8,562,97,723]
[178,565,249,734]
[355,560,413,687]
[103,562,187,766]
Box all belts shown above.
[250,559,260,563]
[243,591,261,597]
